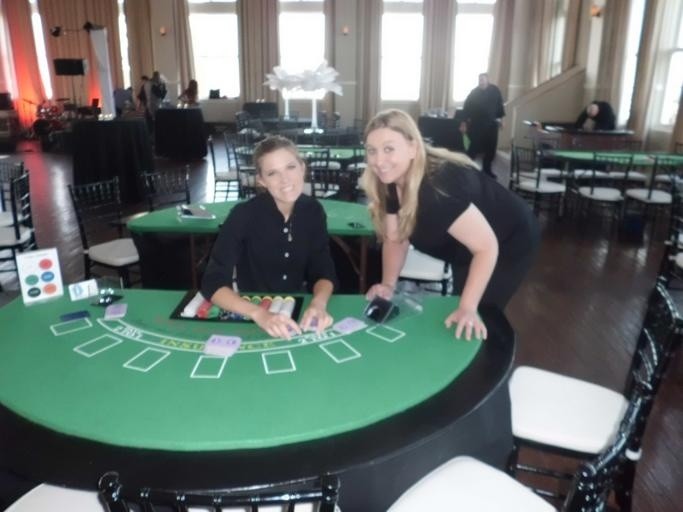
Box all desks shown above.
[1,287,517,512]
[544,146,683,219]
[239,146,365,199]
[127,198,376,295]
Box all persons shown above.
[459,73,505,179]
[581,103,615,130]
[178,80,200,109]
[137,75,149,111]
[360,109,538,342]
[145,71,167,121]
[202,137,337,341]
[115,87,134,117]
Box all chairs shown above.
[311,126,340,188]
[532,138,567,180]
[626,156,683,248]
[347,147,367,188]
[141,164,191,212]
[609,140,647,188]
[73,124,141,206]
[0,160,29,226]
[270,130,298,146]
[208,135,243,202]
[0,170,38,292]
[385,389,643,512]
[655,143,683,191]
[81,116,155,175]
[298,147,341,199]
[67,176,140,288]
[223,132,256,201]
[578,151,636,237]
[311,169,357,204]
[354,118,368,134]
[155,106,207,161]
[347,126,363,145]
[514,145,565,226]
[509,277,682,512]
[508,139,545,192]
[568,142,609,187]
[232,144,267,199]
[98,470,342,512]
